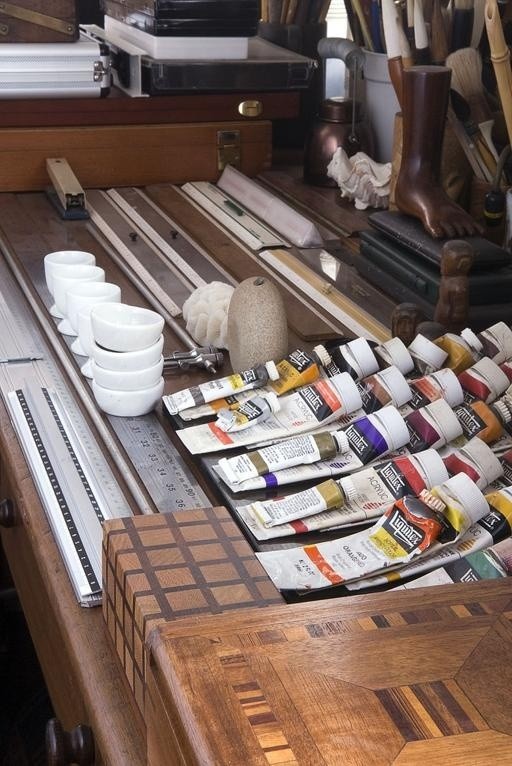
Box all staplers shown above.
[41,155,91,221]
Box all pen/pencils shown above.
[0,357,44,363]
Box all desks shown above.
[1,178,511,765]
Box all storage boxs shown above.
[144,578,511,766]
[100,501,287,727]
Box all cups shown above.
[45,245,165,417]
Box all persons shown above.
[394,65,486,241]
